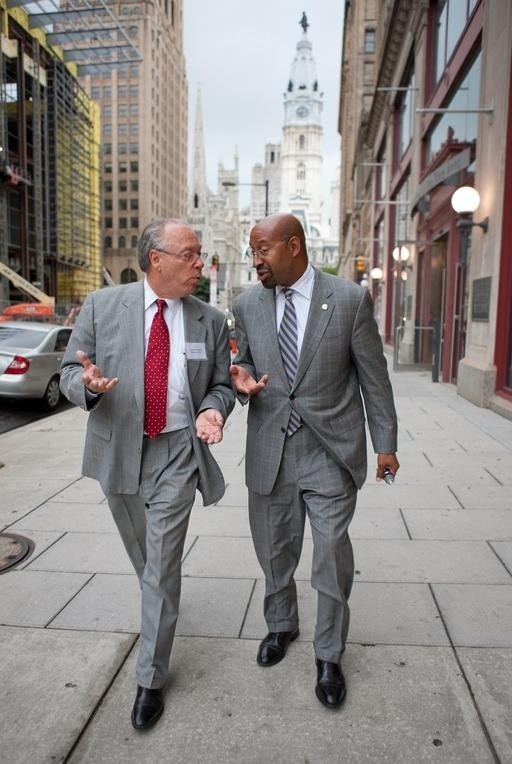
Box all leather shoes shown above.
[256,629,300,667]
[314,658,347,707]
[131,685,164,730]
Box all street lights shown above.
[222,179,268,217]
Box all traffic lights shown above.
[356,256,364,273]
[211,254,219,270]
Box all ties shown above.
[278,288,299,438]
[145,298,169,438]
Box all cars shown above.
[0,322,73,410]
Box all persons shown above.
[229,213,399,708]
[59,219,235,729]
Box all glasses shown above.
[156,248,208,265]
[245,239,289,259]
